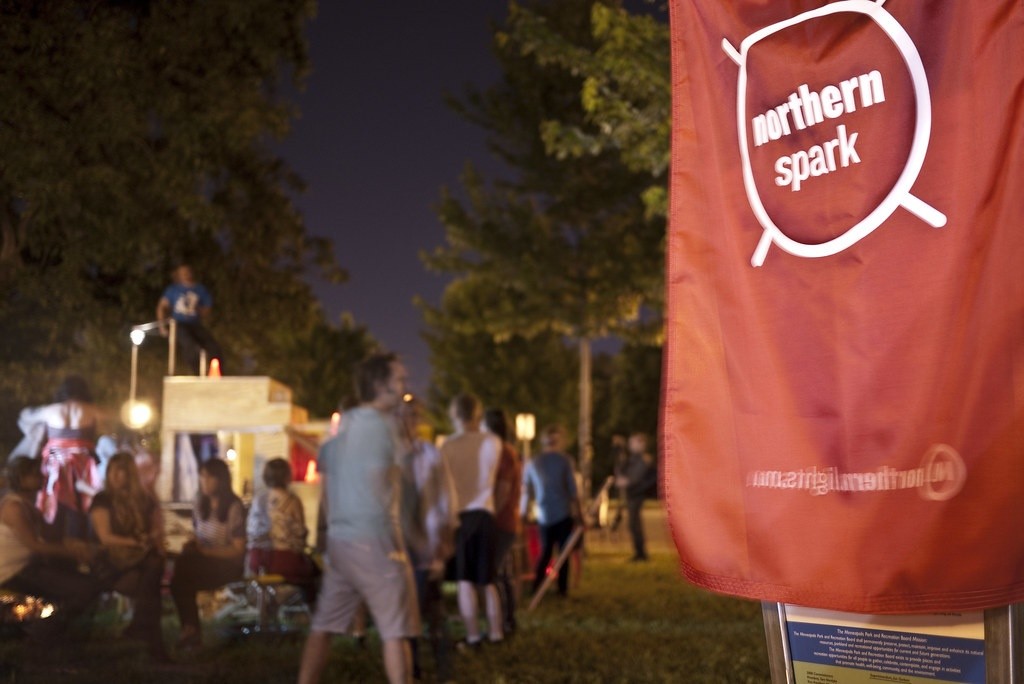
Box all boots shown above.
[124,620,175,664]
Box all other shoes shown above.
[455,636,481,655]
[175,631,203,649]
[481,634,505,647]
[502,619,516,636]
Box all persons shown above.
[298,355,586,684]
[156,263,224,376]
[616,433,656,562]
[0,377,323,646]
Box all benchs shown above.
[78,570,288,648]
